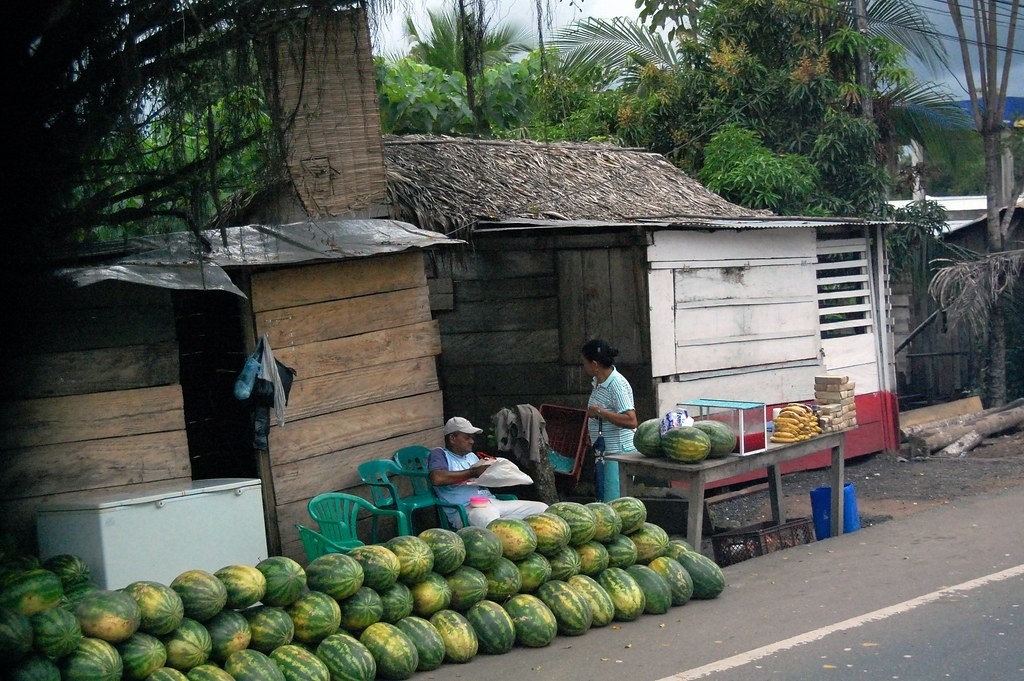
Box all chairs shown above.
[308,492,408,548]
[294,524,386,563]
[359,459,447,543]
[394,445,519,531]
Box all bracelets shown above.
[472,467,476,476]
[596,410,600,415]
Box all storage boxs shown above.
[676,398,768,457]
[711,516,812,568]
[539,404,588,489]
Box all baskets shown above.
[711,514,815,569]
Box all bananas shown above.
[770,403,821,442]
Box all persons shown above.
[580,340,637,502]
[427,416,549,529]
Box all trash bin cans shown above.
[810,483,861,541]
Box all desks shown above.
[605,424,860,549]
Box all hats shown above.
[444,416,483,436]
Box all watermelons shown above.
[633,418,664,457]
[0,495,723,681]
[693,420,736,458]
[662,427,711,463]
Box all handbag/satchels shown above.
[250,340,297,408]
[455,451,534,489]
[235,337,262,400]
